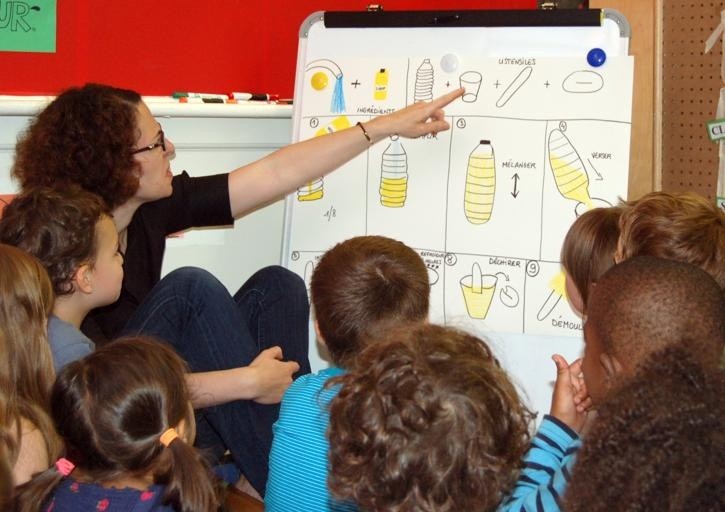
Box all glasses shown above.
[130,124,166,154]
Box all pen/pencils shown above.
[172,91,293,106]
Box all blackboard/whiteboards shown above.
[1,0,591,118]
[281,10,630,440]
